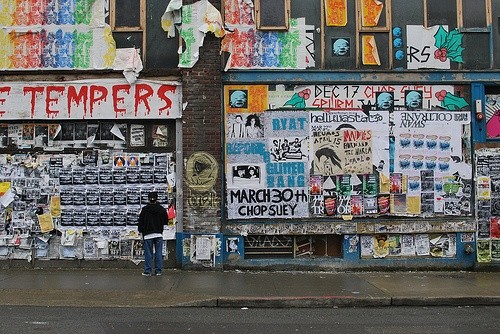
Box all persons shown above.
[137,191,169,277]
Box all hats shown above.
[149,192,158,203]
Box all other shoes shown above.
[155,271,161,275]
[141,272,151,276]
